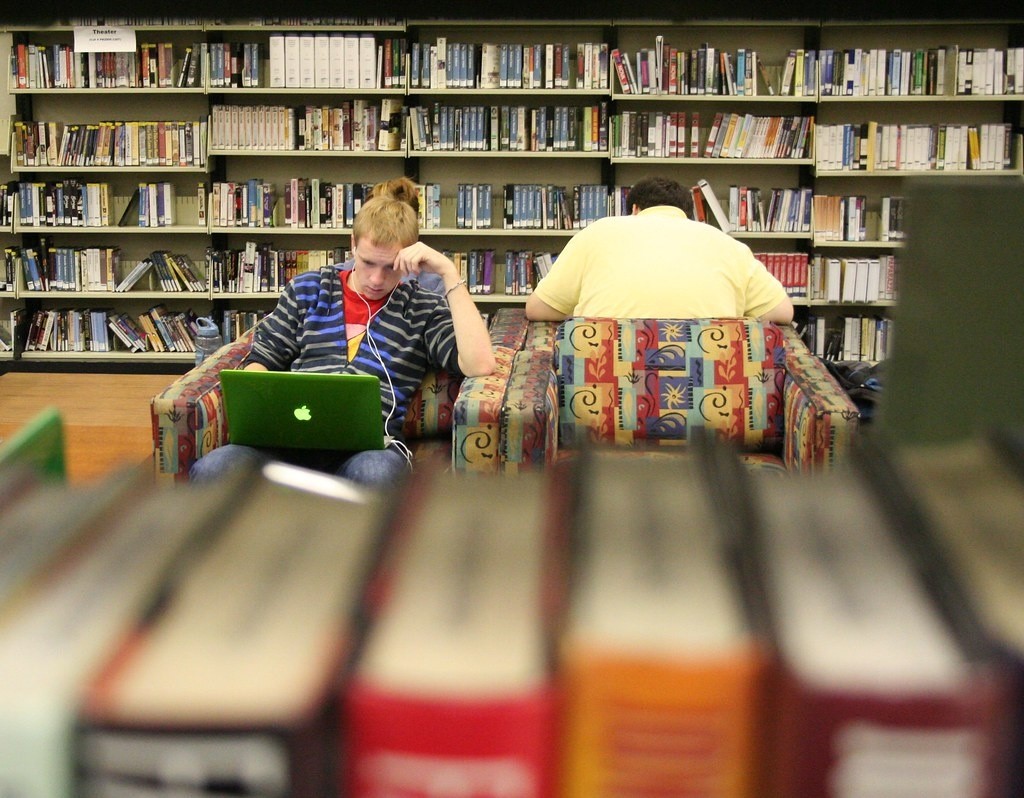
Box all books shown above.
[4,439,1024,798]
[0,17,1024,362]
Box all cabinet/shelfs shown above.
[0,0,1024,798]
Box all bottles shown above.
[195,317,223,367]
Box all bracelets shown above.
[444,280,467,297]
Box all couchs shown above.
[524,319,815,358]
[501,320,858,487]
[150,343,514,492]
[236,305,528,349]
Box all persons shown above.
[188,177,495,502]
[525,176,795,325]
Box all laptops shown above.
[220,368,395,452]
